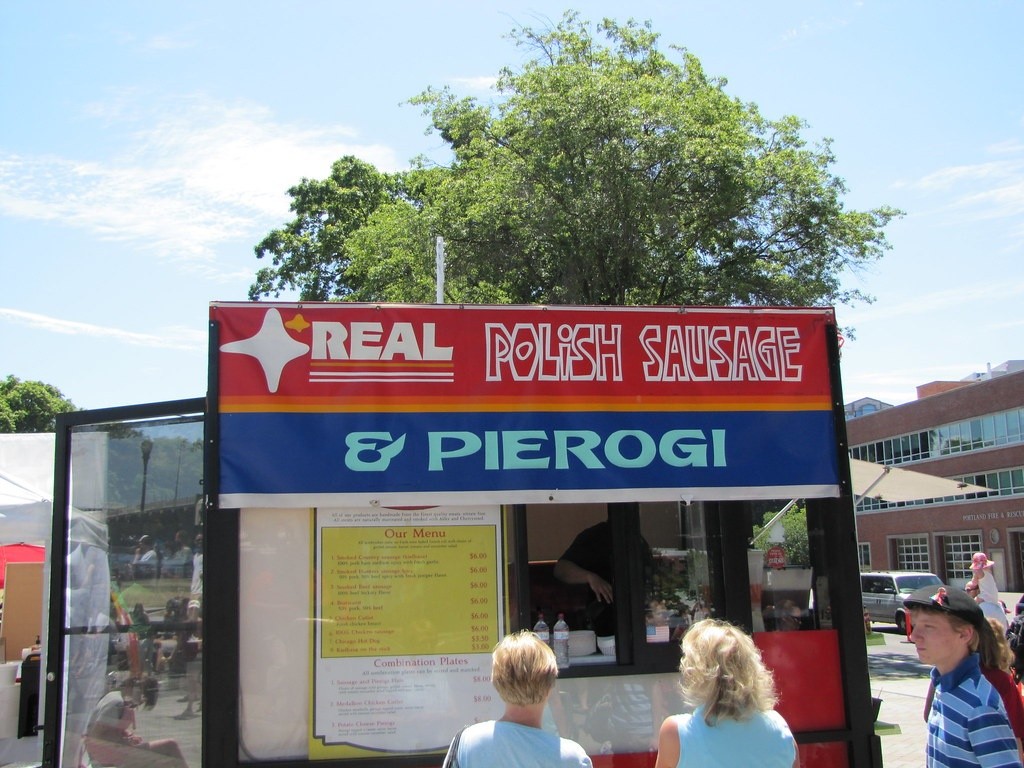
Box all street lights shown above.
[136,440,153,537]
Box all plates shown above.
[569,630,596,656]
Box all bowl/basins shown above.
[597,636,616,656]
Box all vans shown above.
[859,570,945,634]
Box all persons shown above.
[904,586,1024,768]
[441,629,594,768]
[965,553,999,605]
[554,503,653,653]
[966,584,1009,637]
[84,676,189,768]
[976,617,1024,766]
[66,528,204,689]
[654,618,801,768]
[166,601,202,720]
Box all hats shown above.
[969,552,995,570]
[902,585,985,632]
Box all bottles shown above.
[535,614,550,645]
[553,613,569,669]
